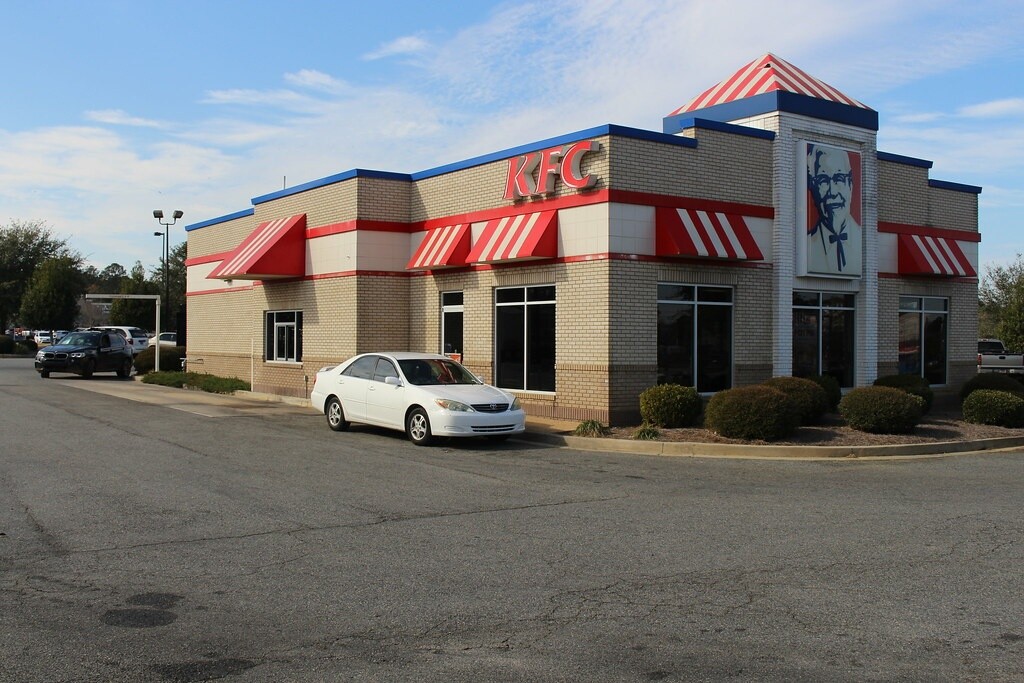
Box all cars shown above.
[148,331,176,348]
[310,350,525,446]
[3,326,69,345]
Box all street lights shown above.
[153,231,164,292]
[152,208,182,330]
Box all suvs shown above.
[33,329,134,378]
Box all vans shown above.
[81,325,148,359]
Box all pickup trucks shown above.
[977,338,1024,374]
